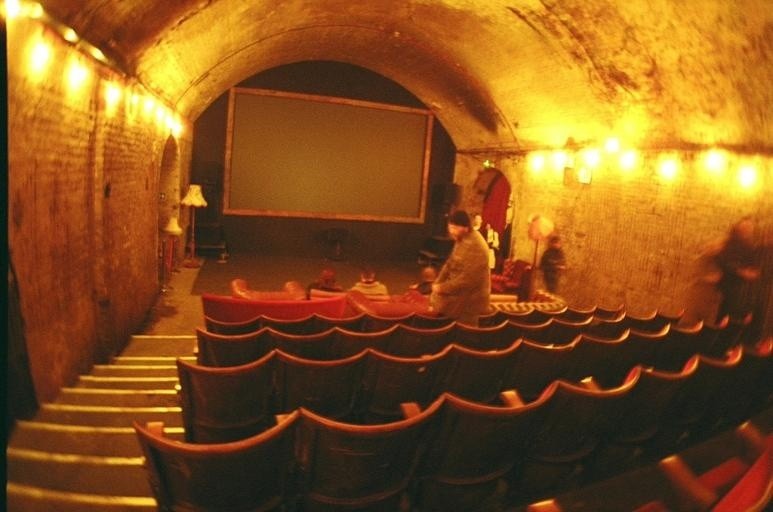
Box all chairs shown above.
[131,260,773,512]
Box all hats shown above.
[448,211,470,227]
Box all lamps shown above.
[527,213,552,269]
[161,217,183,282]
[178,183,211,267]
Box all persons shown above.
[408,266,436,294]
[540,234,569,294]
[347,268,390,295]
[485,221,501,269]
[472,212,487,241]
[306,265,342,299]
[430,210,492,327]
[680,215,773,344]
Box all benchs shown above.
[415,248,438,267]
